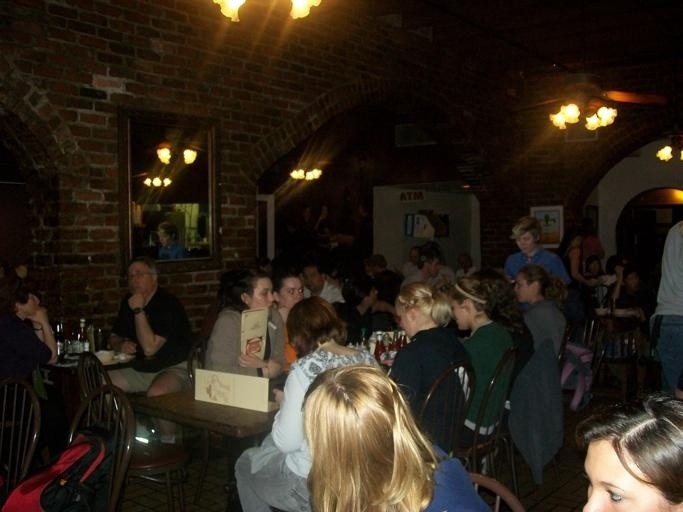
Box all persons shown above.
[156,220,185,259]
[570,390,683,512]
[101,257,194,444]
[648,221,683,392]
[1,283,68,479]
[203,202,646,510]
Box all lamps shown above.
[143,138,198,189]
[549,91,619,130]
[655,134,682,162]
[289,168,323,181]
[212,0,321,22]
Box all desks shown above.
[126,392,280,511]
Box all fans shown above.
[508,58,679,130]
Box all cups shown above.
[93,328,107,352]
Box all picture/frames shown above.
[529,206,564,248]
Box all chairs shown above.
[0,352,188,512]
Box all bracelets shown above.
[131,307,144,314]
[33,328,42,331]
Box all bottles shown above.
[347,326,407,366]
[55,318,90,364]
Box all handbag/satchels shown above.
[1,428,114,512]
[561,343,595,394]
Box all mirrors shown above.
[118,106,224,272]
